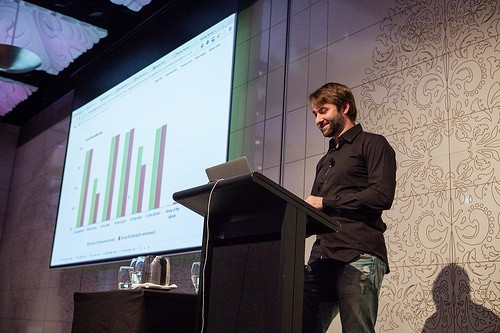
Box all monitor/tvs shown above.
[205,157,252,182]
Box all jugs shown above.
[149,256,171,286]
[143,255,154,282]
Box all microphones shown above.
[328,158,334,166]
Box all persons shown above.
[300,83,397,333]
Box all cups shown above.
[135,256,145,283]
[191,261,200,294]
[118,266,134,290]
[129,258,139,284]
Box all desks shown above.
[71,290,201,333]
